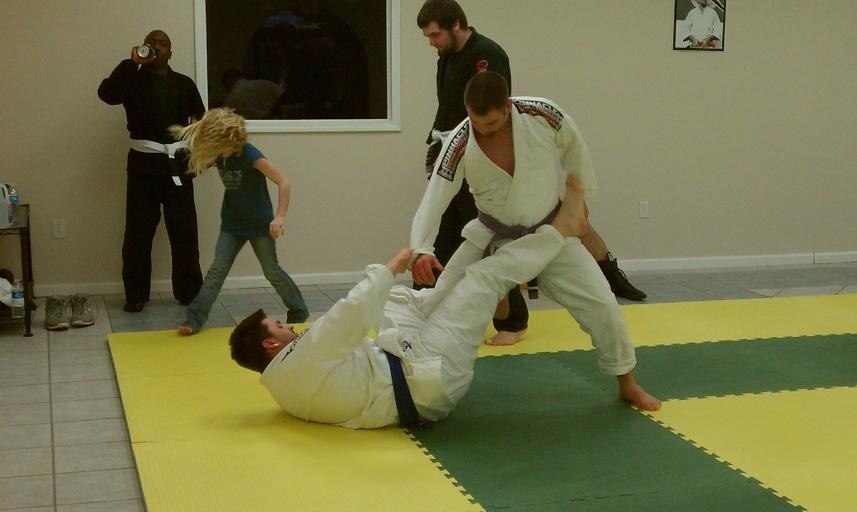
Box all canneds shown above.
[137,45,156,59]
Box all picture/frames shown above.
[191,0,400,134]
[672,0,728,52]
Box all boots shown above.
[599,257,648,301]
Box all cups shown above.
[0,184,11,228]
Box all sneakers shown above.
[42,293,99,331]
[121,300,145,314]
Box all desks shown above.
[0,202,38,337]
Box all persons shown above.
[226,173,587,431]
[406,70,663,413]
[416,0,529,346]
[221,0,369,120]
[94,29,206,314]
[166,102,312,336]
[673,1,723,49]
[578,215,646,301]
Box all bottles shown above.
[6,184,19,224]
[10,277,25,318]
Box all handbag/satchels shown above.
[0,180,19,230]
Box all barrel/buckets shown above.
[1,183,14,229]
